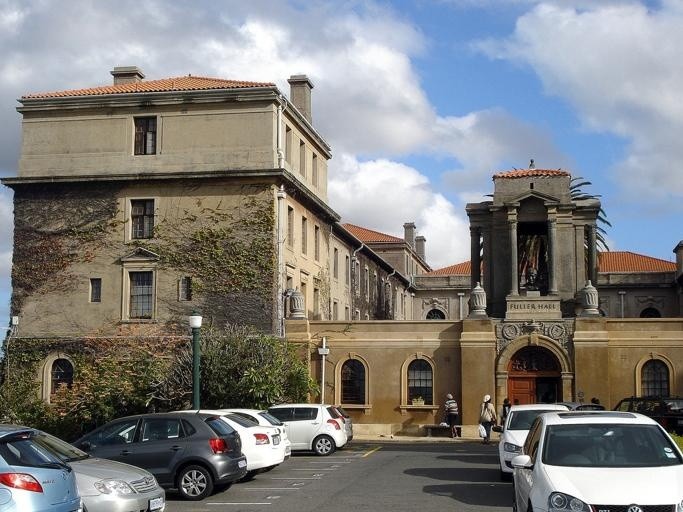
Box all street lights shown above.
[187,309,203,410]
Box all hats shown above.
[447,393,453,400]
[482,394,491,403]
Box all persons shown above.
[499,397,511,425]
[477,394,496,442]
[520,267,541,287]
[442,392,459,438]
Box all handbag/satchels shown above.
[490,418,496,425]
[478,424,487,438]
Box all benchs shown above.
[421,423,461,438]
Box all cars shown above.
[491,395,683,511]
